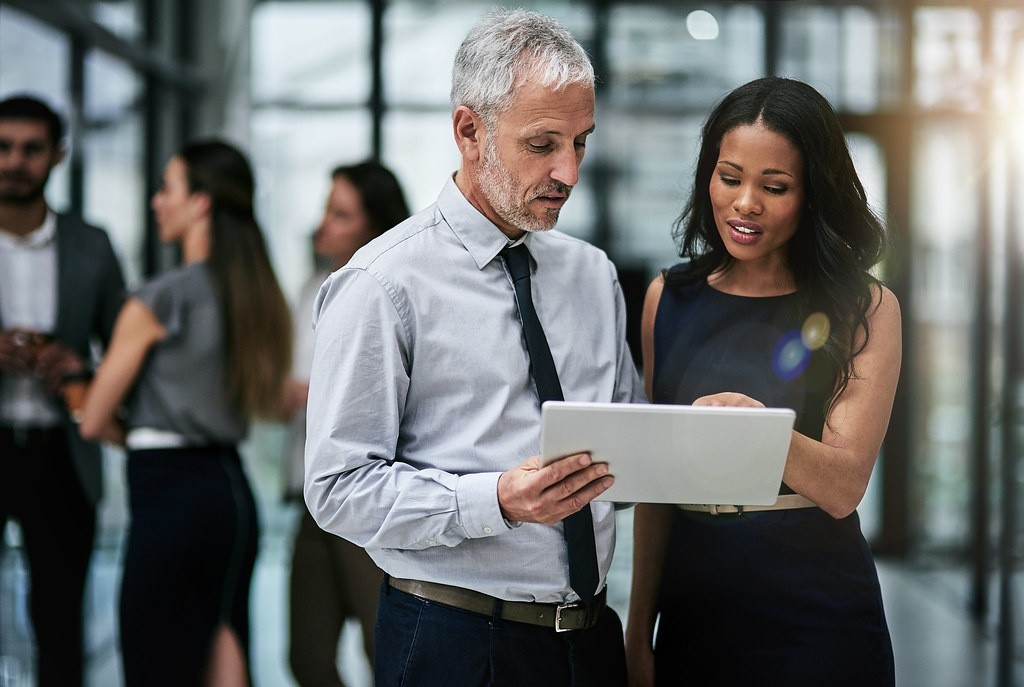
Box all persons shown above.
[300,8,651,687]
[77,136,293,686]
[1,91,132,687]
[623,76,904,687]
[286,158,414,687]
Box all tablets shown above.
[540,401,796,506]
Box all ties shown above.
[498,242,600,604]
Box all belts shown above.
[387,574,599,633]
[678,494,820,516]
